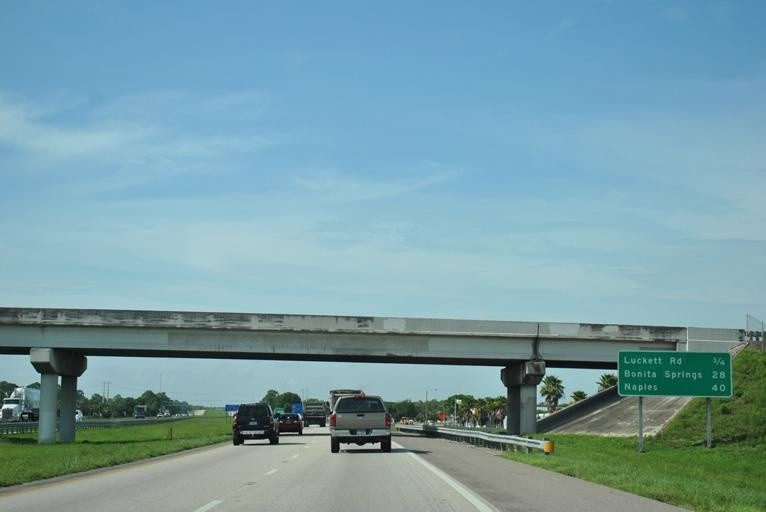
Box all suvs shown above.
[328,393,394,452]
[231,402,280,446]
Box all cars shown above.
[278,413,303,435]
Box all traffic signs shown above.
[616,350,733,399]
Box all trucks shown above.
[303,399,327,427]
[1,386,41,422]
